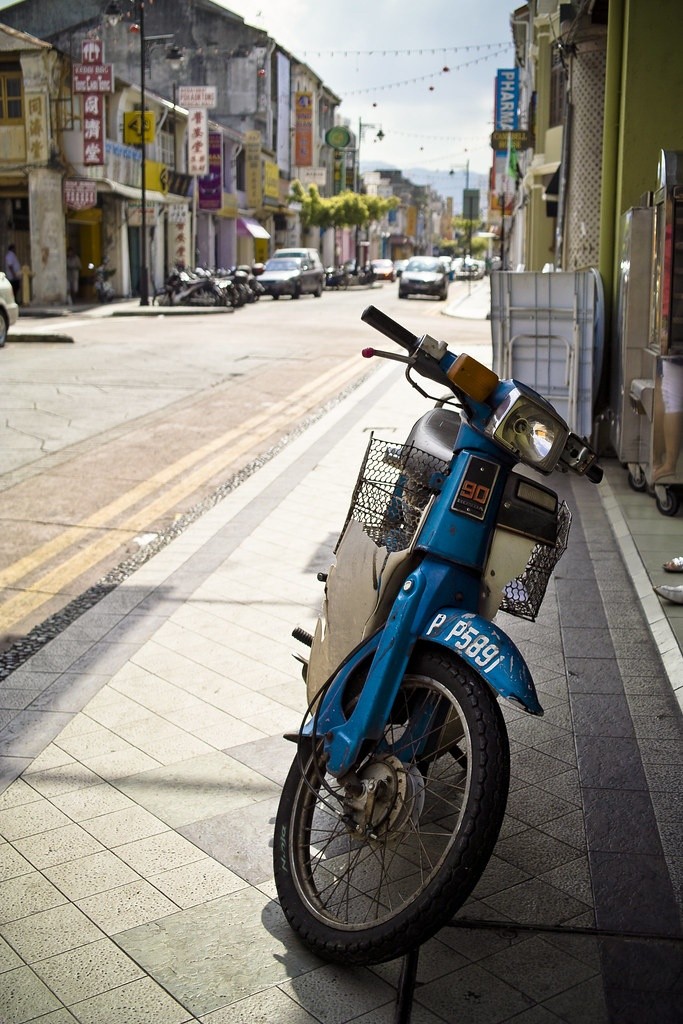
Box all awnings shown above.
[236,217,271,239]
[66,175,191,204]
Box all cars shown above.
[343,258,361,275]
[366,259,398,283]
[439,256,488,280]
[257,256,325,300]
[0,272,19,348]
[395,259,408,277]
[398,258,450,301]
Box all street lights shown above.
[448,160,473,257]
[355,115,384,273]
[138,42,186,309]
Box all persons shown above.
[651,583,683,605]
[67,246,81,304]
[653,360,683,483]
[4,244,22,304]
[662,556,683,573]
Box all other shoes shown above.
[653,584,683,605]
[661,556,683,572]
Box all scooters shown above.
[153,258,265,308]
[271,305,651,970]
[90,255,117,304]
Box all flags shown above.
[507,137,524,182]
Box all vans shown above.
[273,248,323,271]
